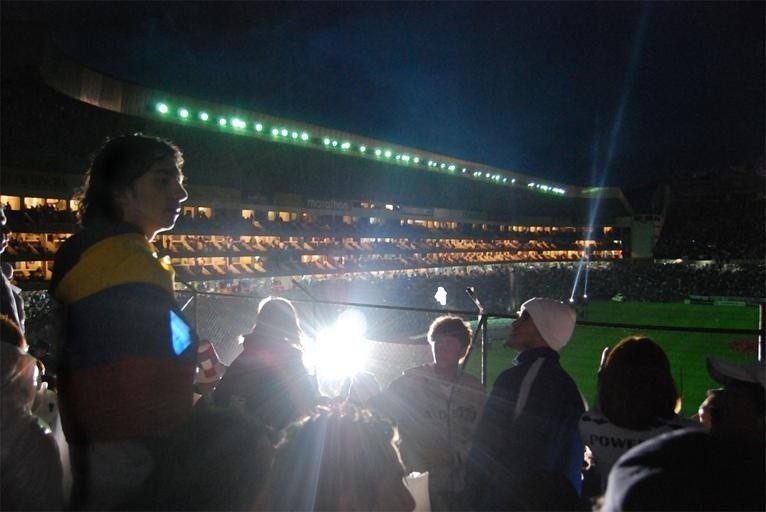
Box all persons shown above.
[0,190,765,512]
[45,130,201,508]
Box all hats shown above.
[707,356,765,401]
[522,296,577,352]
